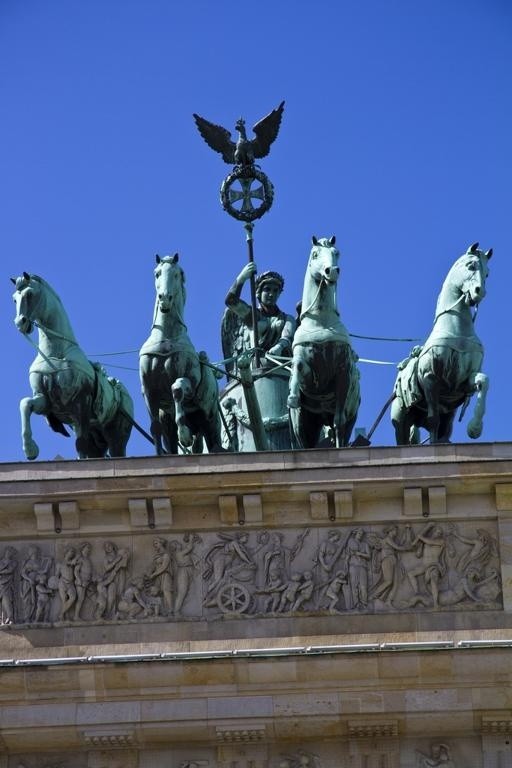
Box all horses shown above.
[391,242,494,445]
[138,252,226,455]
[286,235,360,449]
[9,271,134,461]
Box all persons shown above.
[225,263,295,370]
